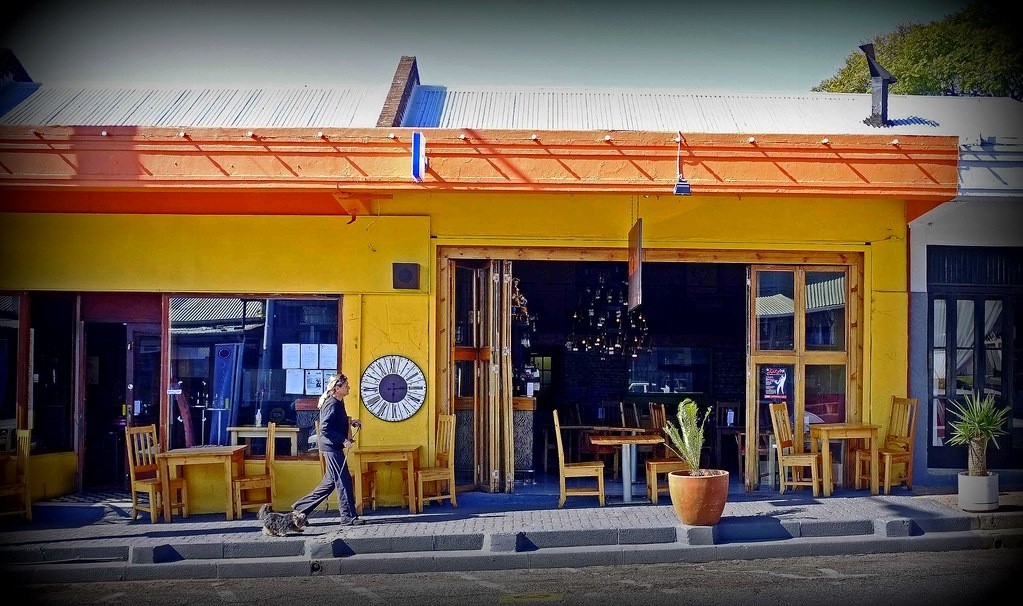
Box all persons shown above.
[289,374,366,526]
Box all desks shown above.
[155,444,248,522]
[807,424,880,497]
[588,435,662,507]
[352,444,420,513]
[227,425,300,457]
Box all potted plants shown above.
[662,397,729,526]
[943,388,1013,512]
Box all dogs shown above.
[259,502,309,538]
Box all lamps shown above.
[673,131,692,197]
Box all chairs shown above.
[0,430,33,519]
[716,396,920,495]
[316,422,377,515]
[400,413,455,513]
[233,423,277,520]
[125,423,188,521]
[543,399,689,508]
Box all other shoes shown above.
[341,516,364,525]
